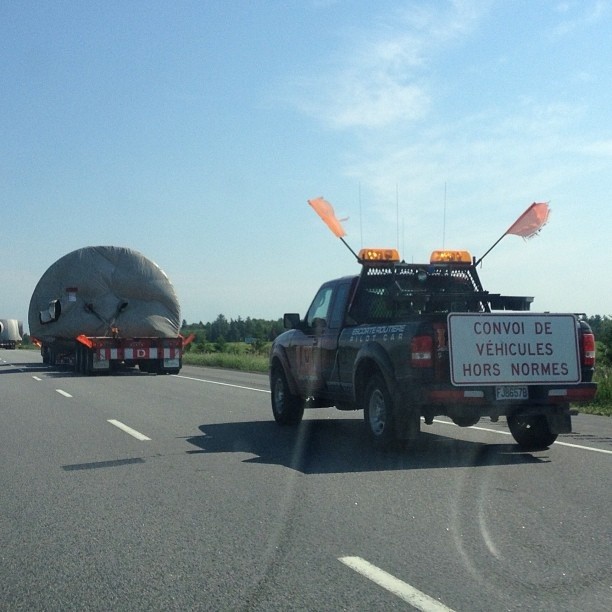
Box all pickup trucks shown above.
[269,247,599,450]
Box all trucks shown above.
[28,244,182,376]
